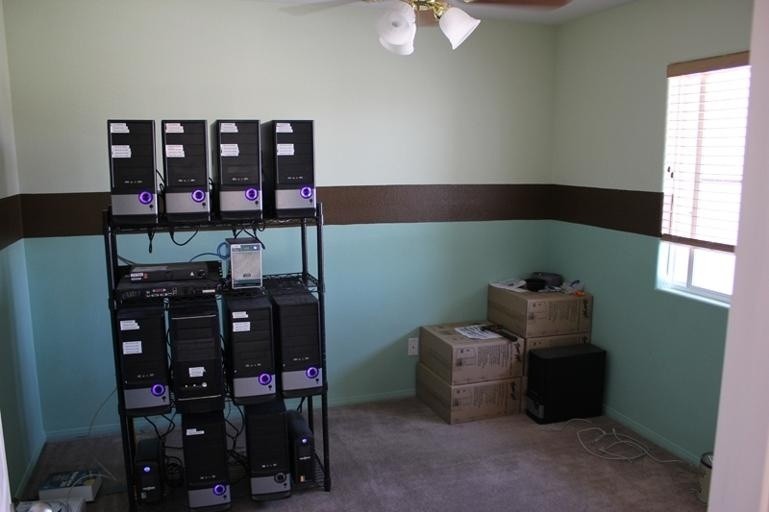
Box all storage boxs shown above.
[415,281,593,425]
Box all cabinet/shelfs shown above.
[101,202,331,512]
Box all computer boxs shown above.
[107,119,324,511]
[526,343,606,424]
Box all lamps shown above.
[378,0,482,55]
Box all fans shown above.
[279,0,571,28]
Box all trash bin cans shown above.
[695,452,714,504]
